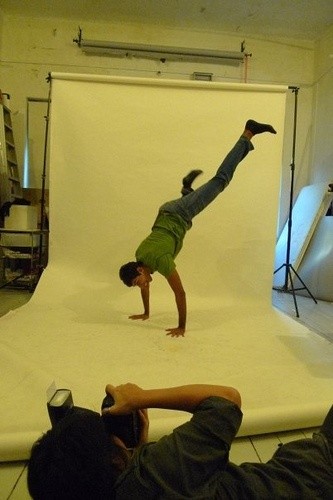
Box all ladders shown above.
[0,103,23,202]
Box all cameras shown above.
[46,389,141,449]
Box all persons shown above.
[28,383,333,500]
[119,120,278,339]
